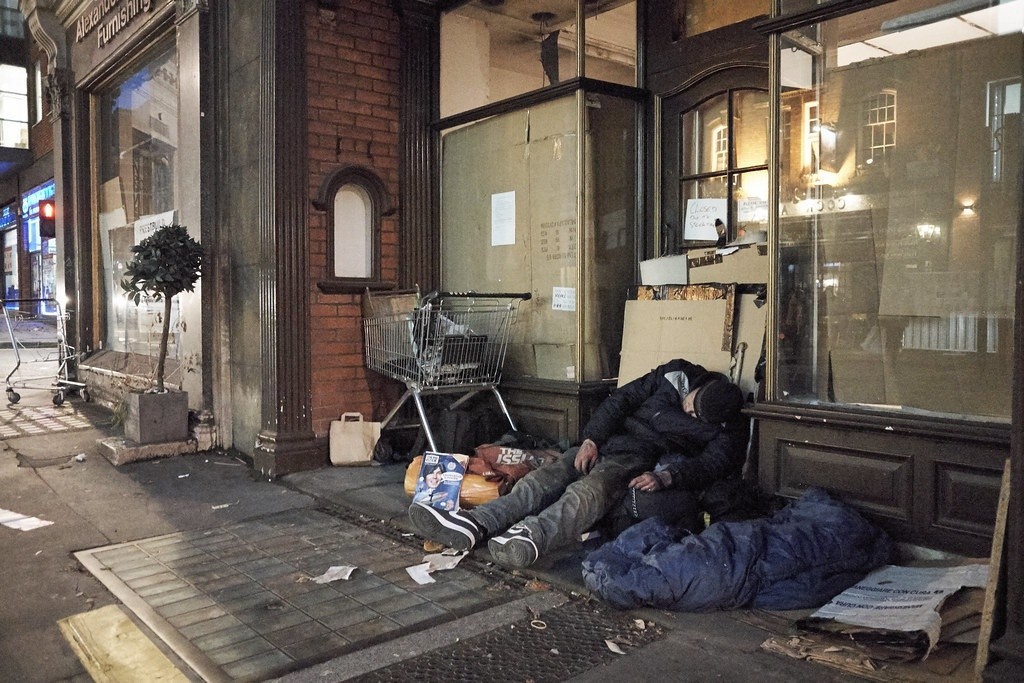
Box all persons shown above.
[424,463,446,488]
[409,360,745,567]
[8,285,16,299]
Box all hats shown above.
[693,380,744,423]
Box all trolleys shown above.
[365,291,531,462]
[1,299,91,405]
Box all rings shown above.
[648,489,652,492]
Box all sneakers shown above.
[407,502,484,551]
[488,526,539,569]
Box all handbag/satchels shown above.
[329,412,381,467]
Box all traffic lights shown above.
[39,200,55,238]
